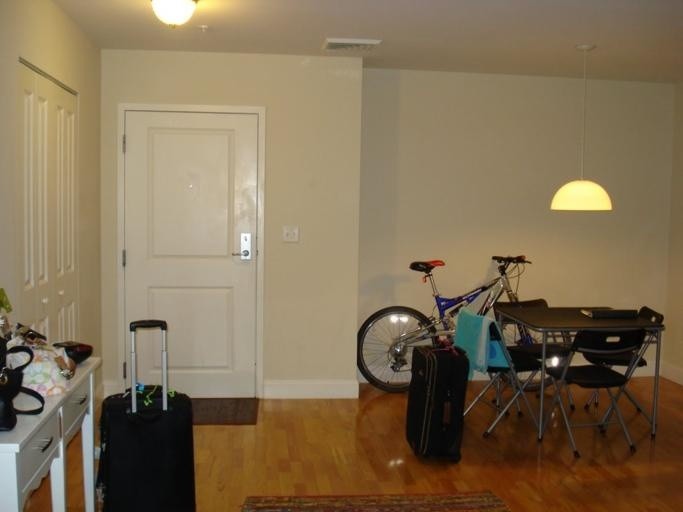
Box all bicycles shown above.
[356,251,572,393]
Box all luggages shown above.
[406,341,469,464]
[95,318,195,512]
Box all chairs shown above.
[461,298,665,459]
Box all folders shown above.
[580,308,638,319]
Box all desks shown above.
[0,356,104,512]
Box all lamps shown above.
[150,0,199,30]
[550,44,614,211]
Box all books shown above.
[579,306,638,320]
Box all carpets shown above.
[240,488,511,512]
[190,398,260,425]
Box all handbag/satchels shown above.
[1,345,45,430]
[6,327,75,397]
